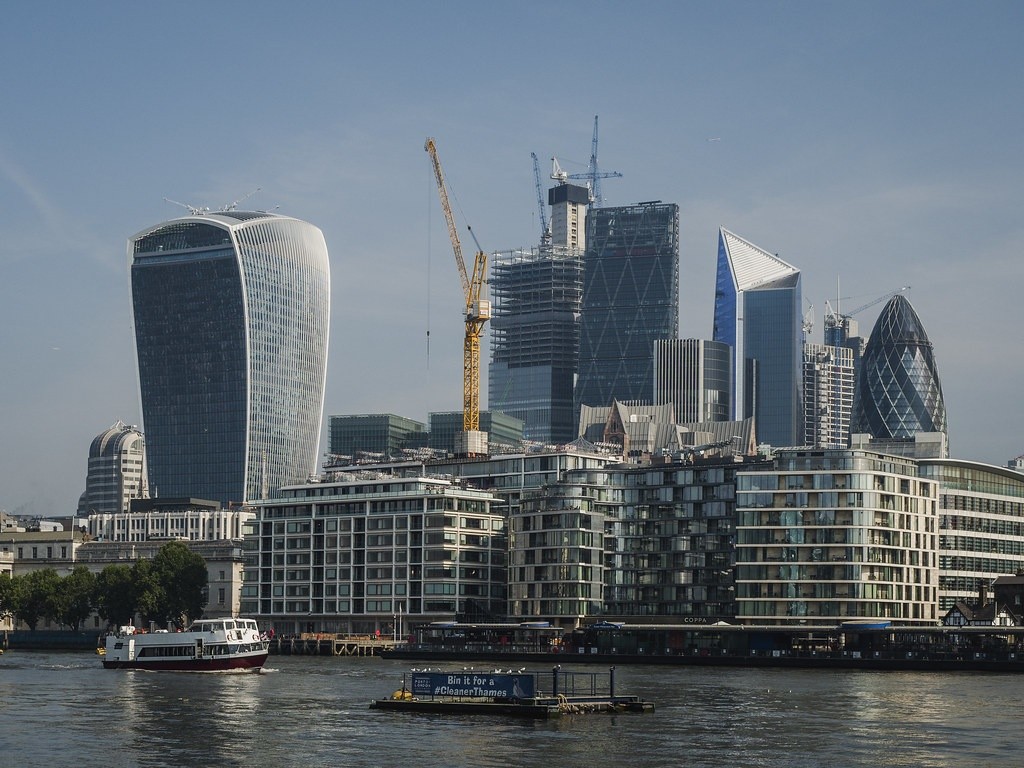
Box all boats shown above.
[101,615,272,675]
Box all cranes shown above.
[527,110,624,253]
[425,136,494,460]
[803,286,912,356]
